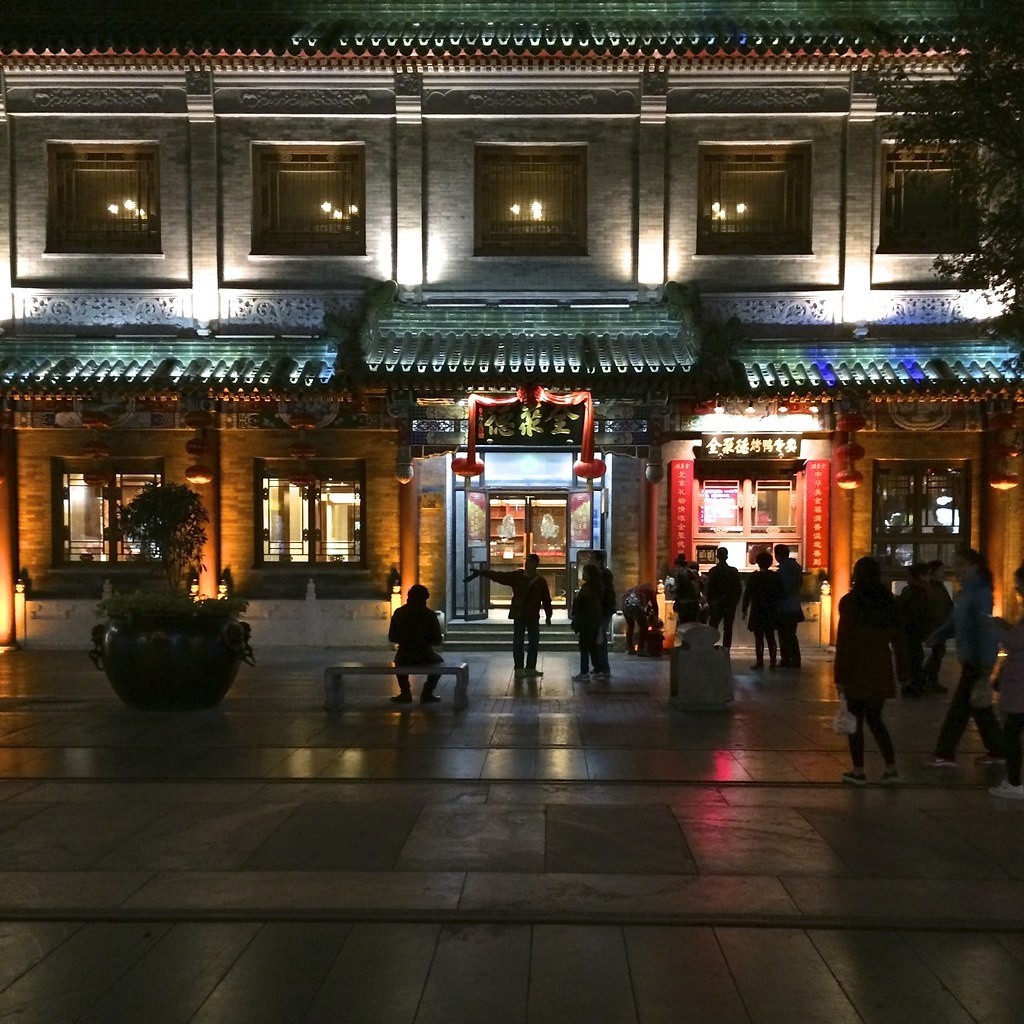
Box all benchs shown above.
[323,662,470,712]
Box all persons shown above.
[388,585,444,703]
[987,564,1024,800]
[921,549,1009,767]
[570,564,616,681]
[834,556,906,784]
[673,544,803,668]
[622,583,659,656]
[469,554,552,676]
[895,561,953,696]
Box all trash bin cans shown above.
[669,622,734,710]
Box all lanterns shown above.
[574,459,606,492]
[184,410,213,494]
[451,456,484,486]
[81,410,113,498]
[835,412,865,500]
[987,413,1020,499]
[289,412,317,495]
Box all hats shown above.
[674,552,700,570]
[927,560,943,571]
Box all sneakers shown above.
[880,767,899,785]
[975,749,1006,766]
[921,752,957,769]
[987,779,1024,800]
[841,771,866,785]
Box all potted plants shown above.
[88,481,256,712]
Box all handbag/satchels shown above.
[833,692,858,736]
[798,606,804,623]
[508,597,525,619]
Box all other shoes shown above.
[781,662,801,672]
[572,669,611,683]
[920,676,949,692]
[625,649,652,657]
[769,663,776,673]
[390,692,412,703]
[905,682,921,699]
[514,668,544,679]
[420,693,441,703]
[750,662,764,670]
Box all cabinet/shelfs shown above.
[490,504,526,537]
